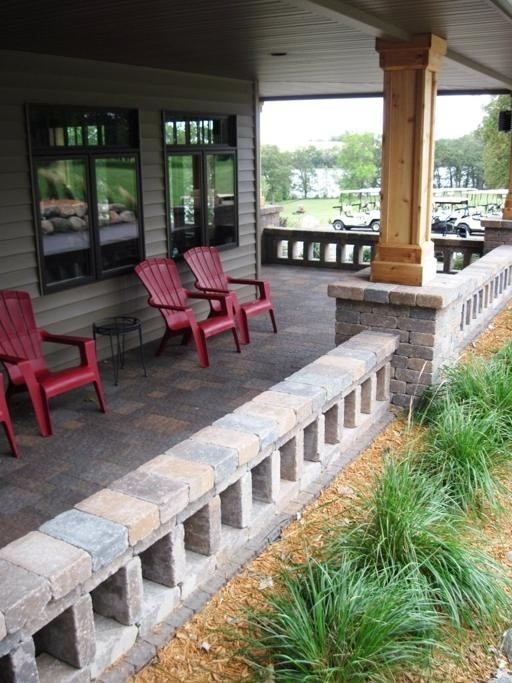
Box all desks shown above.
[91,313,149,385]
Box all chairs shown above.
[134,257,241,367]
[0,373,19,461]
[182,246,277,343]
[0,290,106,435]
